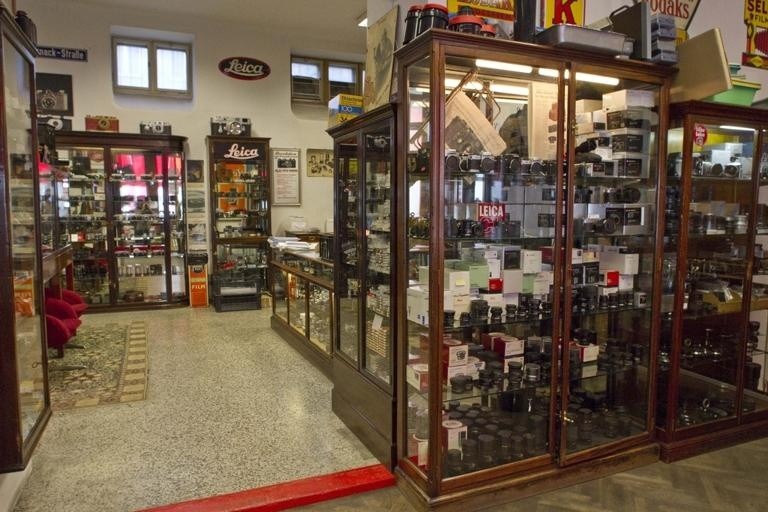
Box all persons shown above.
[134,199,153,256]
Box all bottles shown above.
[118,264,148,277]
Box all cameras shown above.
[37,89,68,112]
[585,216,617,234]
[86,115,118,133]
[462,221,484,237]
[212,116,250,136]
[70,173,184,304]
[38,117,71,132]
[365,132,597,175]
[215,173,268,265]
[665,152,740,177]
[141,121,171,136]
[497,221,520,238]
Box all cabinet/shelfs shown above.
[268,238,333,381]
[327,22,764,508]
[51,123,271,312]
[0,30,74,470]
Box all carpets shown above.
[48,319,147,409]
